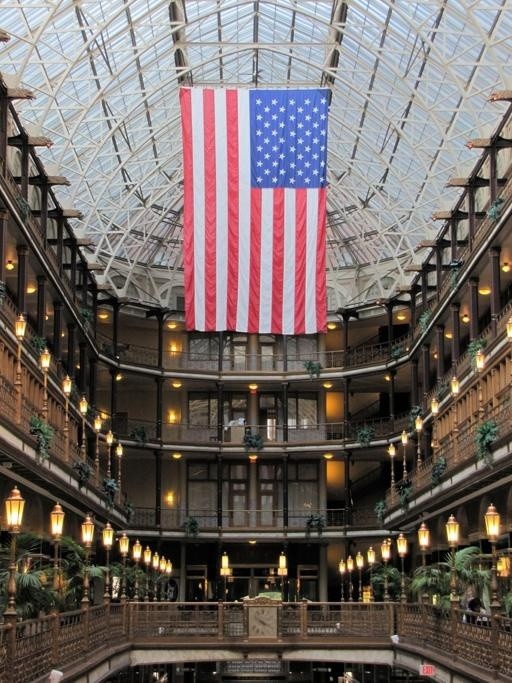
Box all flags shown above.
[180,87,331,336]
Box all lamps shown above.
[3,485,171,614]
[12,313,123,479]
[336,503,502,609]
[386,315,485,486]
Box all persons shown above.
[462,599,491,629]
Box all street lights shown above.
[219,552,231,602]
[0,313,124,485]
[277,551,289,602]
[334,504,500,614]
[386,317,512,483]
[3,485,174,627]
